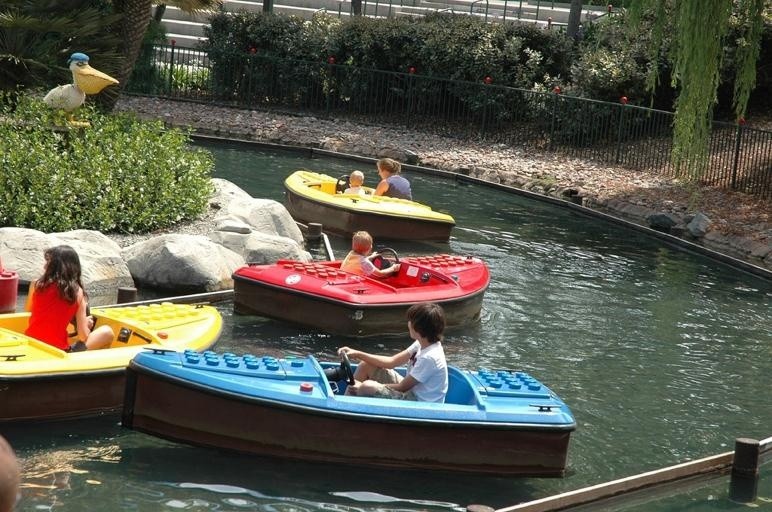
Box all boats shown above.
[121,347,577,478]
[0,301,225,425]
[231,248,491,339]
[285,170,456,245]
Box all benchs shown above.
[444,366,477,404]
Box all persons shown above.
[337,302,449,403]
[337,170,366,195]
[22,245,115,354]
[0,432,20,512]
[339,231,401,277]
[368,158,412,200]
